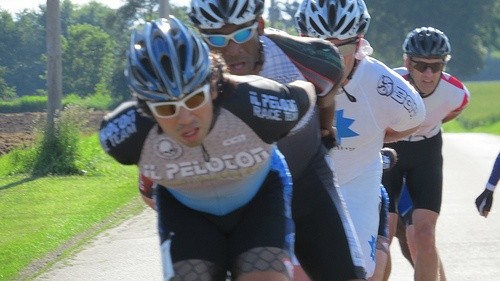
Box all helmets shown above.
[402,26,451,57]
[123,15,210,101]
[186,0,264,29]
[294,0,371,40]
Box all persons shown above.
[294,0,426,281]
[376,27,469,281]
[99,14,317,281]
[475,151,500,217]
[187,0,369,281]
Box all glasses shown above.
[199,21,260,48]
[144,81,211,119]
[410,58,445,73]
[337,38,360,58]
